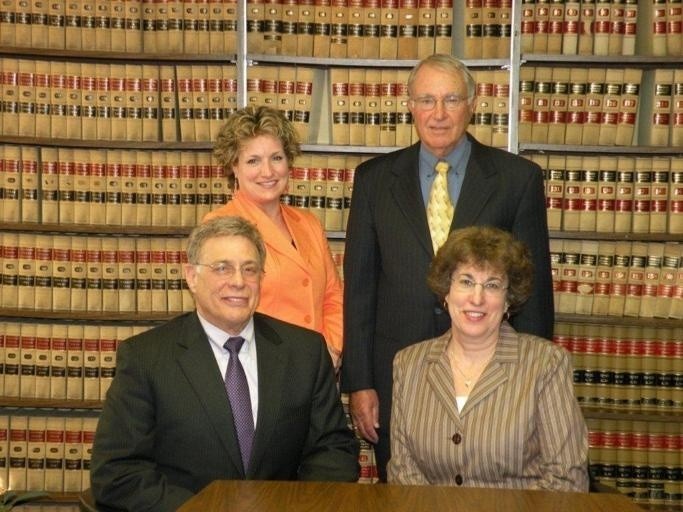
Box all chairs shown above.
[70,480,100,510]
[588,476,622,495]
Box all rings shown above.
[353,425,357,430]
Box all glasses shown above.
[449,275,513,298]
[191,260,267,283]
[408,92,473,111]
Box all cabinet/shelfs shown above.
[0,1,681,510]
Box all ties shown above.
[425,162,457,256]
[224,337,254,476]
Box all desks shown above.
[170,473,647,510]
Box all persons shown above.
[200,103,344,395]
[341,51,555,484]
[384,224,592,495]
[90,214,363,511]
